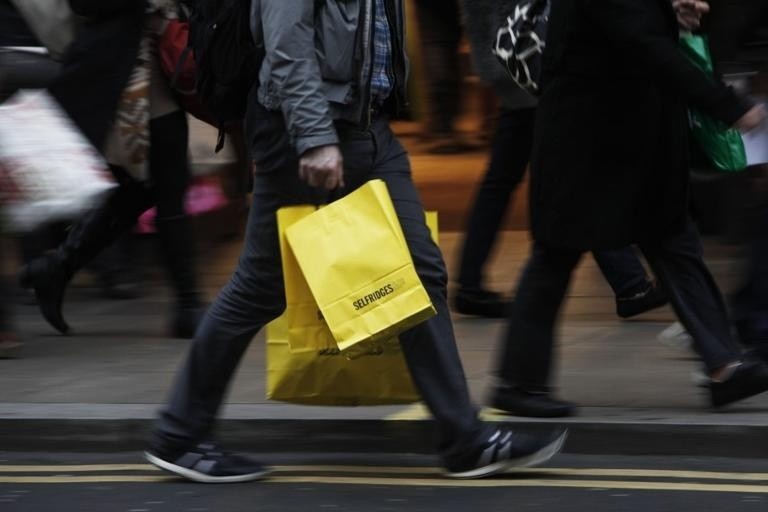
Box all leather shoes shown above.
[489,383,576,418]
[708,363,767,408]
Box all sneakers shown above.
[143,433,267,486]
[439,422,568,479]
[614,280,672,319]
[450,277,519,318]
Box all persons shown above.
[0,0,256,341]
[143,0,572,484]
[405,0,768,318]
[481,2,768,419]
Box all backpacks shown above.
[150,1,253,137]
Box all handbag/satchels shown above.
[265,201,439,405]
[284,178,438,363]
